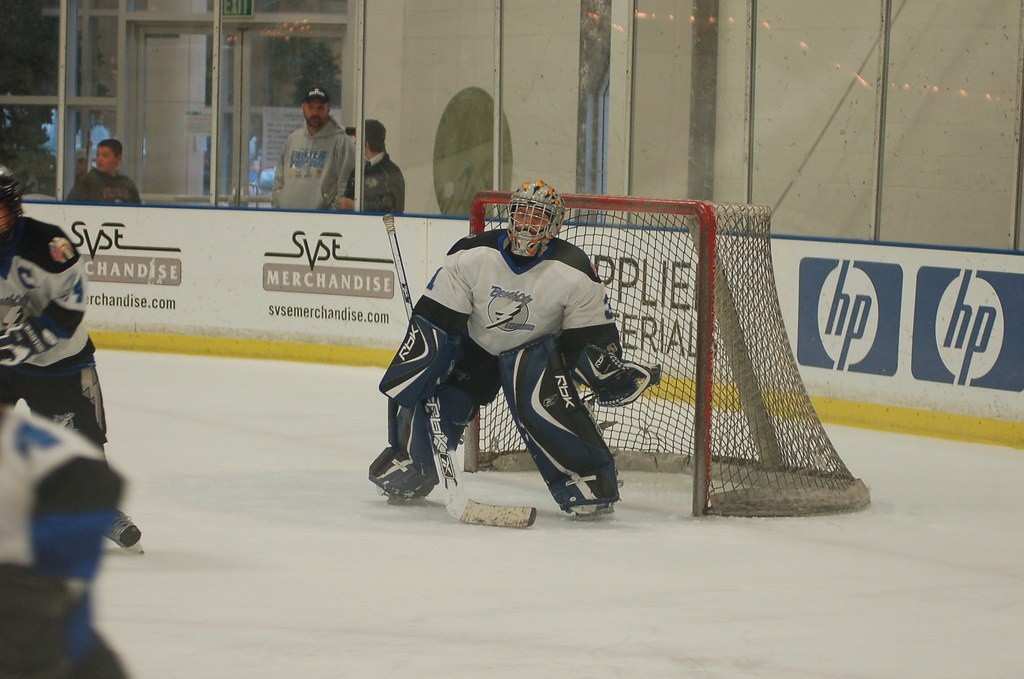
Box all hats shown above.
[301,85,330,104]
[345,120,387,143]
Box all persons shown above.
[1,162,146,553]
[62,138,143,204]
[0,401,131,679]
[197,86,406,213]
[372,177,661,522]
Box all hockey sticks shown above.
[383,214,537,530]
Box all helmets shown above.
[0,165,25,238]
[507,178,565,257]
